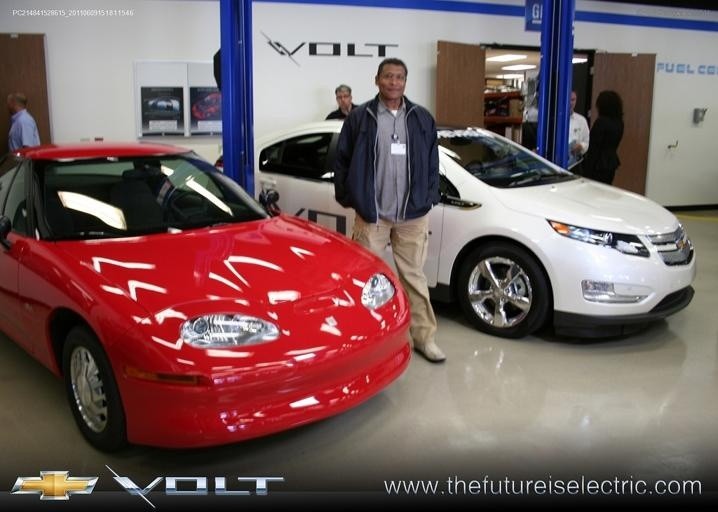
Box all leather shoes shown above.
[414,342,446,362]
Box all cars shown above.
[191,92,221,120]
[211,122,697,342]
[0,135,410,457]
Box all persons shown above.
[589,90,623,185]
[568,91,590,172]
[7,92,40,151]
[326,84,359,119]
[336,58,446,363]
